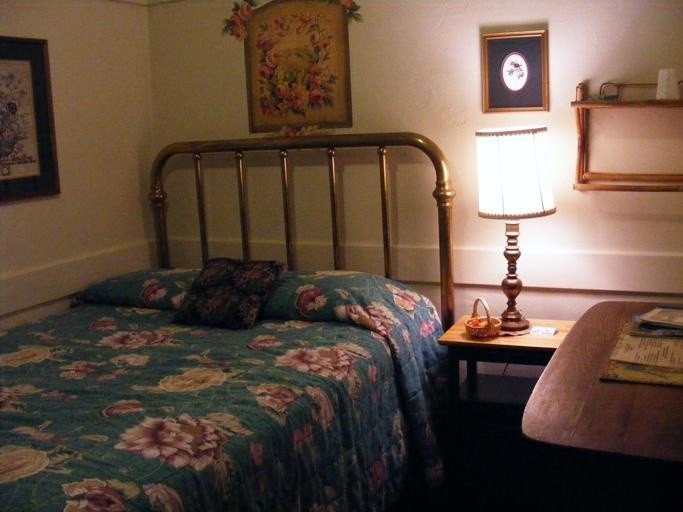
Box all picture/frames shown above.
[479,28,550,114]
[0,35,61,202]
[242,0,352,134]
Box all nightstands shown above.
[438,312,598,445]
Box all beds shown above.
[0,132,453,507]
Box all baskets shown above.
[464,297,502,339]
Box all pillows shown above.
[174,255,285,331]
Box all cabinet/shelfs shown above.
[572,75,683,193]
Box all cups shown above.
[655,68,681,101]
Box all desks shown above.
[519,300,683,464]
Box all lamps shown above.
[474,124,557,333]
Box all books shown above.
[629,312,682,340]
[598,319,682,387]
[635,304,682,331]
